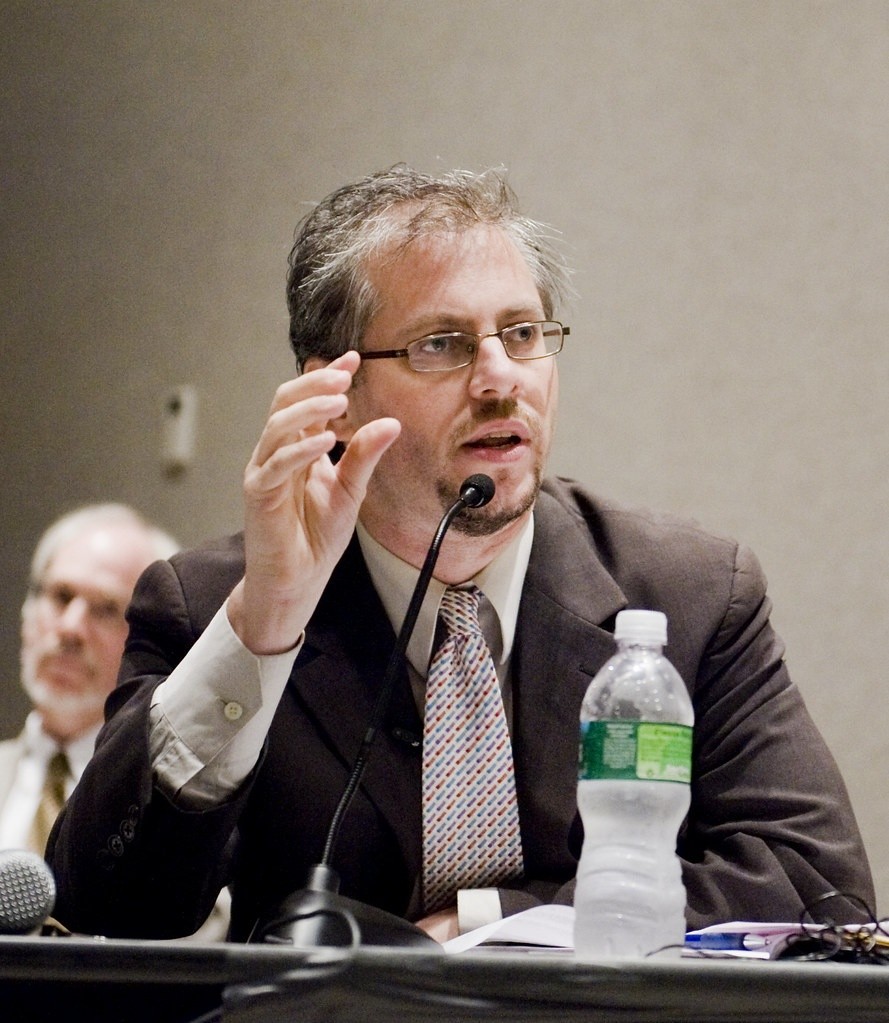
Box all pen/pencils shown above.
[686,930,773,951]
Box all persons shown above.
[43,166,877,942]
[0,504,180,864]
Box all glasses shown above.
[321,320,569,373]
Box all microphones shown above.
[0,848,57,935]
[264,474,495,948]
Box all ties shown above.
[28,753,69,860]
[421,586,524,915]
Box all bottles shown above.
[569,608,700,963]
